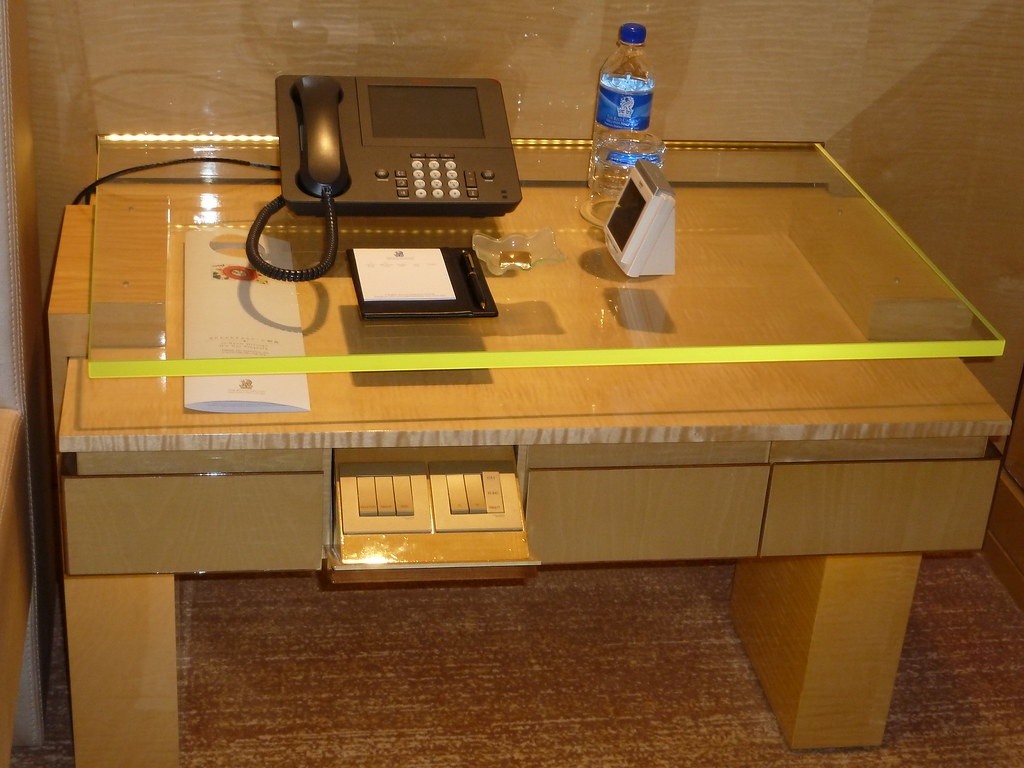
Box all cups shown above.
[579,130,666,228]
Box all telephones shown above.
[276,66,525,218]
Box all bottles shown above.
[587,23,657,198]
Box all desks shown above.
[48,130,1013,768]
